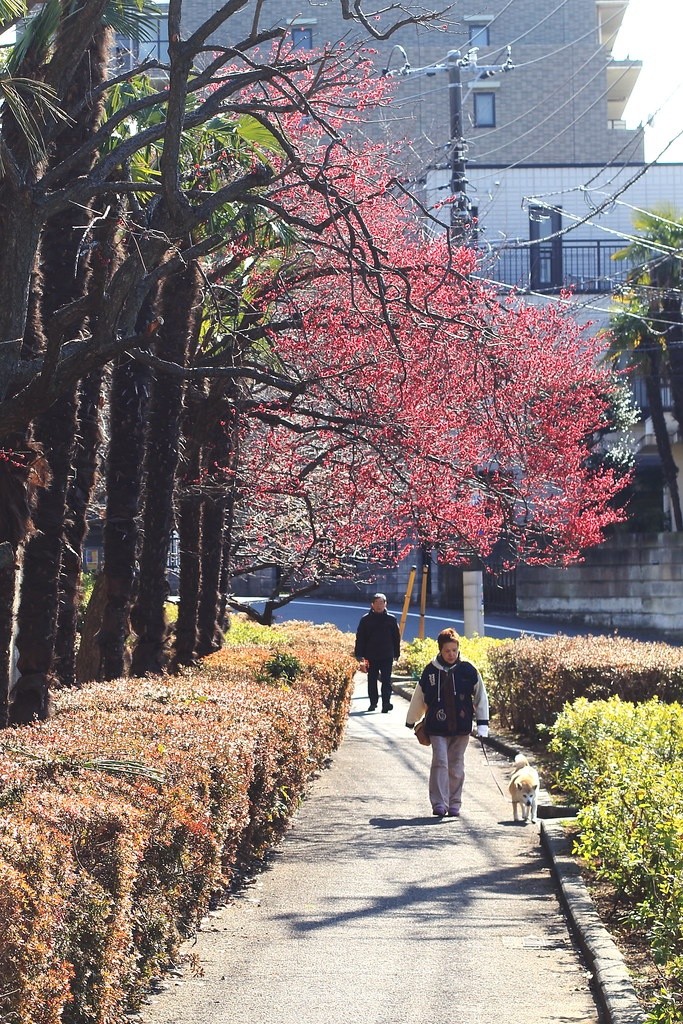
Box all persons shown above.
[354,592,401,713]
[404,628,490,817]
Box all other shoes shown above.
[381,702,393,713]
[433,806,445,816]
[447,806,459,816]
[368,703,376,711]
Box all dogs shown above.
[508,754,540,824]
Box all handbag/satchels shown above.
[415,721,432,747]
